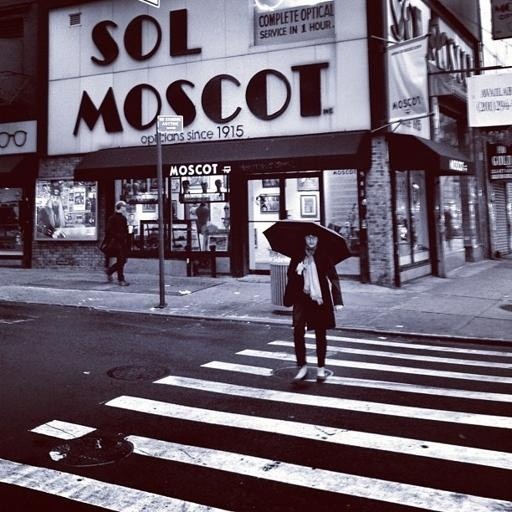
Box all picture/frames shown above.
[204,230,229,251]
[123,178,158,213]
[170,177,211,219]
[296,178,319,216]
[260,179,280,213]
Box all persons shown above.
[104,201,131,287]
[195,202,210,252]
[260,196,268,212]
[179,179,222,195]
[287,228,345,381]
[443,207,454,249]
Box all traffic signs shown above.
[157,114,184,135]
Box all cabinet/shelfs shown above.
[140,218,202,252]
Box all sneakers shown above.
[119,281,129,286]
[105,268,113,280]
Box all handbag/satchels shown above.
[283,280,295,306]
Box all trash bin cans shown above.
[270,261,294,316]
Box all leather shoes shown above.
[317,367,325,380]
[293,366,308,381]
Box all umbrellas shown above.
[263,218,354,276]
